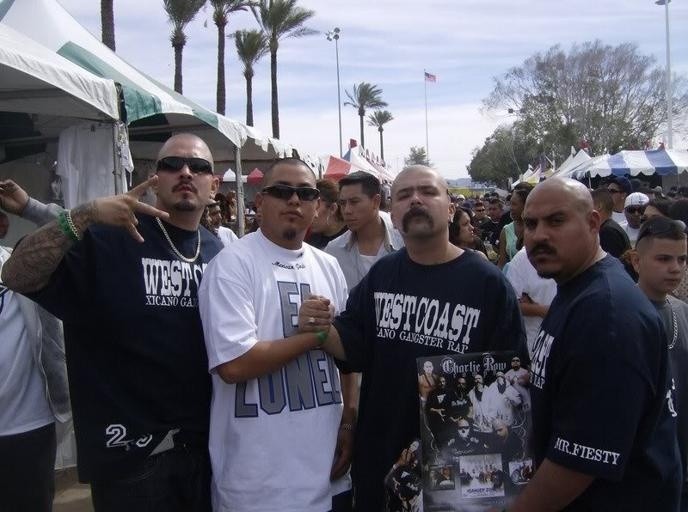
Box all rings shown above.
[308,316,316,325]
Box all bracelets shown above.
[57,211,73,239]
[339,422,352,431]
[315,330,328,343]
[66,210,80,241]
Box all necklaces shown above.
[156,216,201,263]
[666,298,679,350]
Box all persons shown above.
[197,156,358,512]
[322,169,405,297]
[0,177,73,511]
[385,440,421,511]
[510,175,684,511]
[419,356,532,490]
[202,190,239,247]
[1,133,225,512]
[448,180,557,361]
[308,176,349,250]
[629,215,688,511]
[589,175,688,304]
[296,163,530,512]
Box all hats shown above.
[624,192,649,208]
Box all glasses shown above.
[636,219,686,247]
[157,156,213,175]
[627,206,644,213]
[262,185,320,201]
[608,189,622,194]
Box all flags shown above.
[425,72,437,84]
